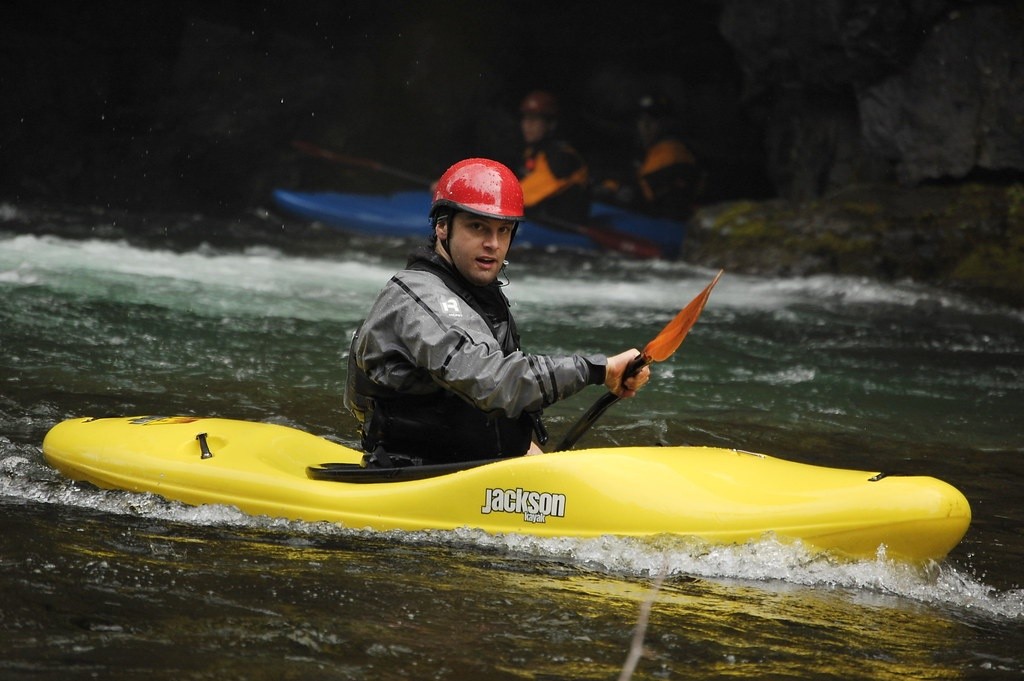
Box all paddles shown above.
[291,138,433,187]
[549,266,726,454]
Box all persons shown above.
[347,159,651,469]
[431,92,592,225]
[596,97,711,216]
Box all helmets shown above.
[632,92,670,117]
[521,93,559,117]
[427,158,526,227]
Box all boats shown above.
[272,185,684,266]
[43,414,972,578]
